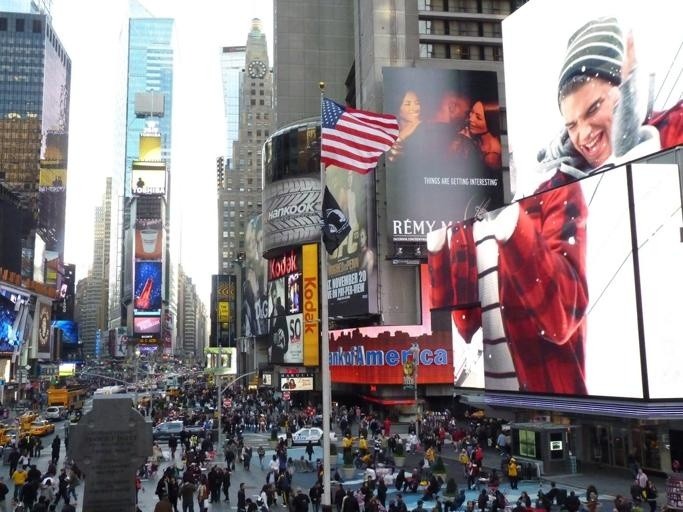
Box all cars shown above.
[0,360,208,451]
[276,427,337,446]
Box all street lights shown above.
[216,344,259,456]
[205,348,212,367]
[134,344,141,410]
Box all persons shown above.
[386,85,503,235]
[533,18,683,195]
[0,366,682,511]
[427,181,588,395]
[137,178,144,187]
[240,170,379,364]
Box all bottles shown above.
[263,375,267,383]
[135,277,155,308]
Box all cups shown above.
[140,229,157,254]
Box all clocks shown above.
[247,60,267,78]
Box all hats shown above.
[557,18,622,114]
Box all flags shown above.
[321,97,400,174]
